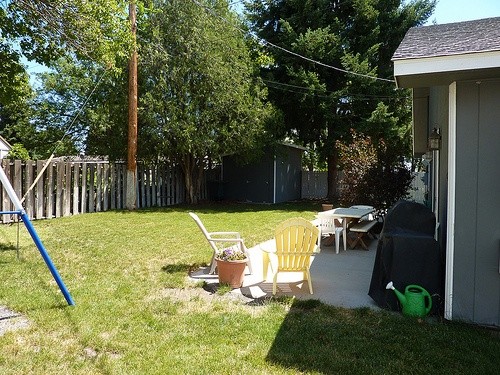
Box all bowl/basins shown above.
[322,204,333,210]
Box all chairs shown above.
[351,205,374,210]
[261,217,319,296]
[317,212,343,254]
[189,212,254,275]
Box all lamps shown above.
[427,128,441,152]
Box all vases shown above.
[216,259,247,288]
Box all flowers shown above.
[220,245,244,259]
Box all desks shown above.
[318,208,374,251]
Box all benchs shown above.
[349,219,377,250]
